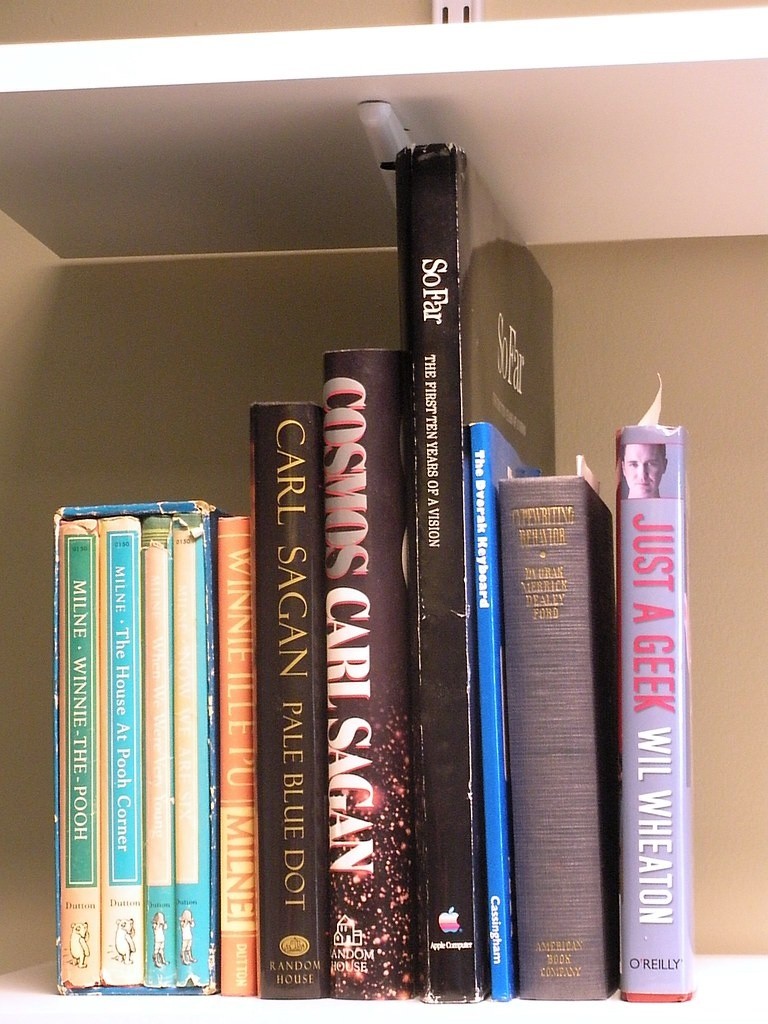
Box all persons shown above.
[621,443,667,498]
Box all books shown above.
[50,140,696,1003]
